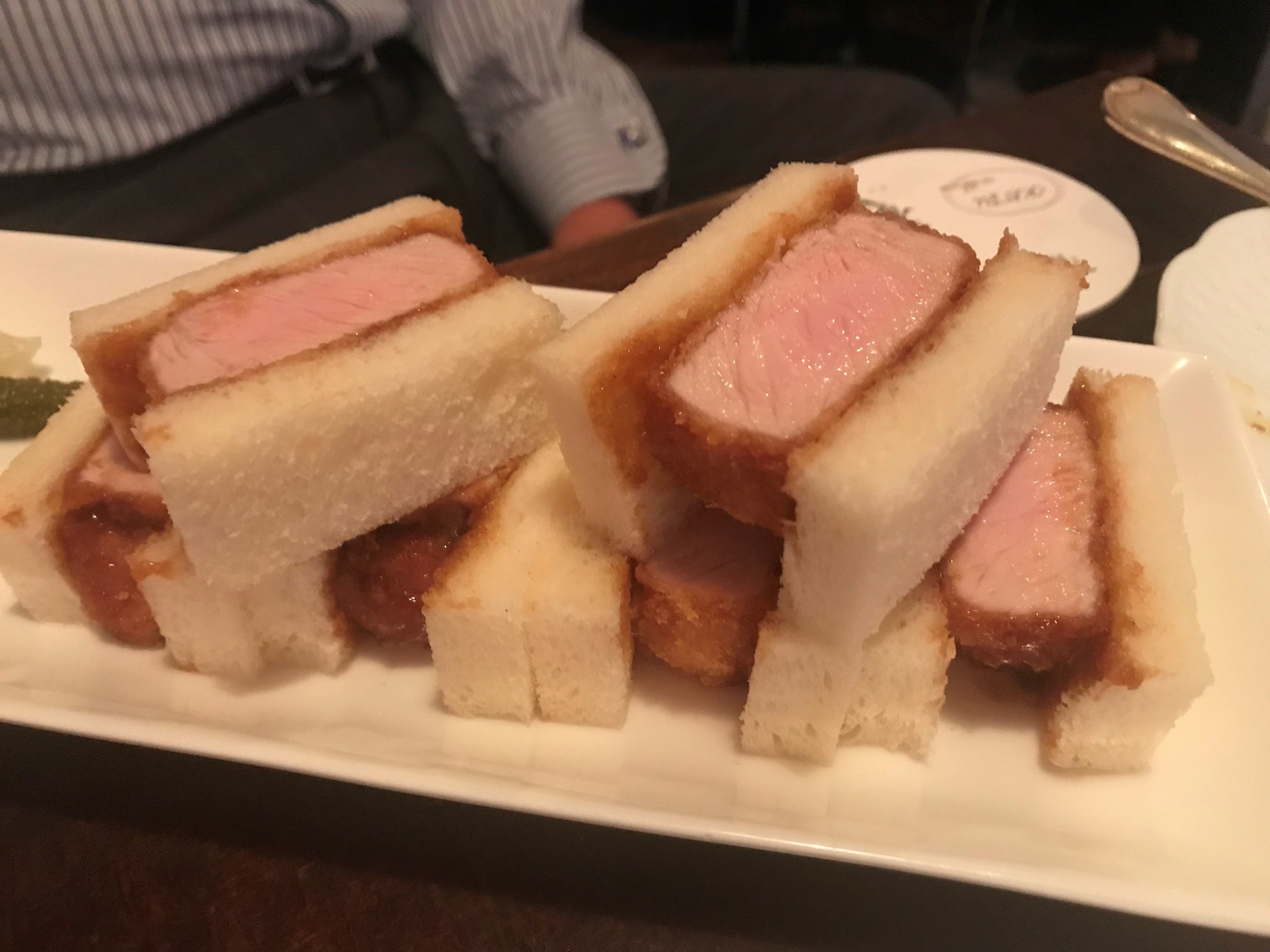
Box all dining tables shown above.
[0,0,1266,952]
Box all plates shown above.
[0,205,1270,939]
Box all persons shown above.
[0,0,666,272]
